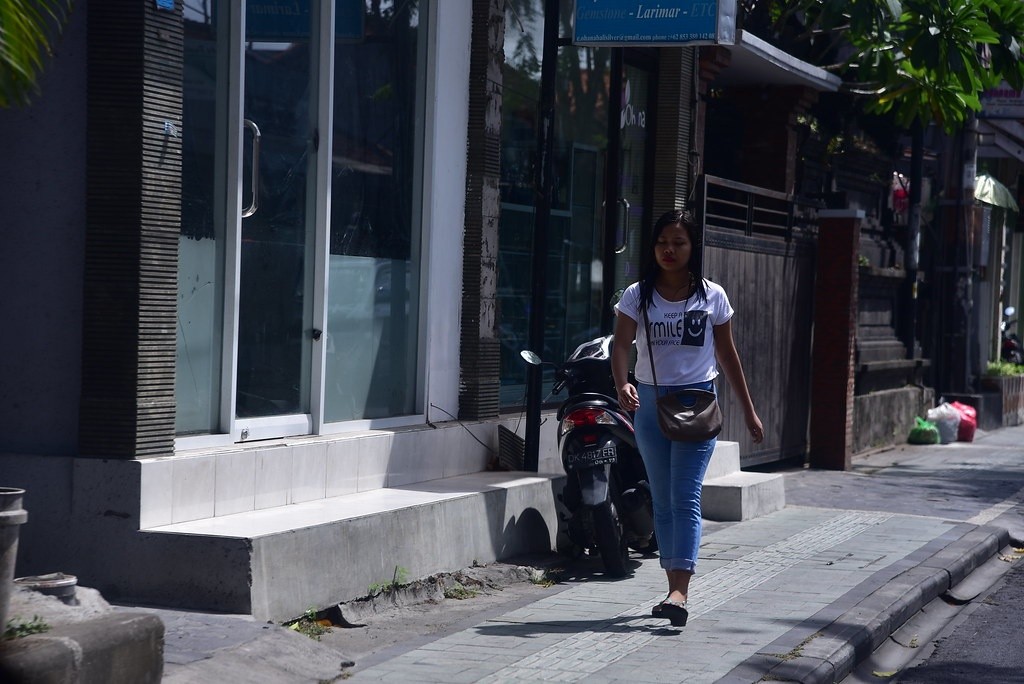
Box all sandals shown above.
[651,597,667,618]
[660,596,689,629]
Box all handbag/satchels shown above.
[655,388,724,443]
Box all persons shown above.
[608,209,767,631]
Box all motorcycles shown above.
[518,288,659,581]
